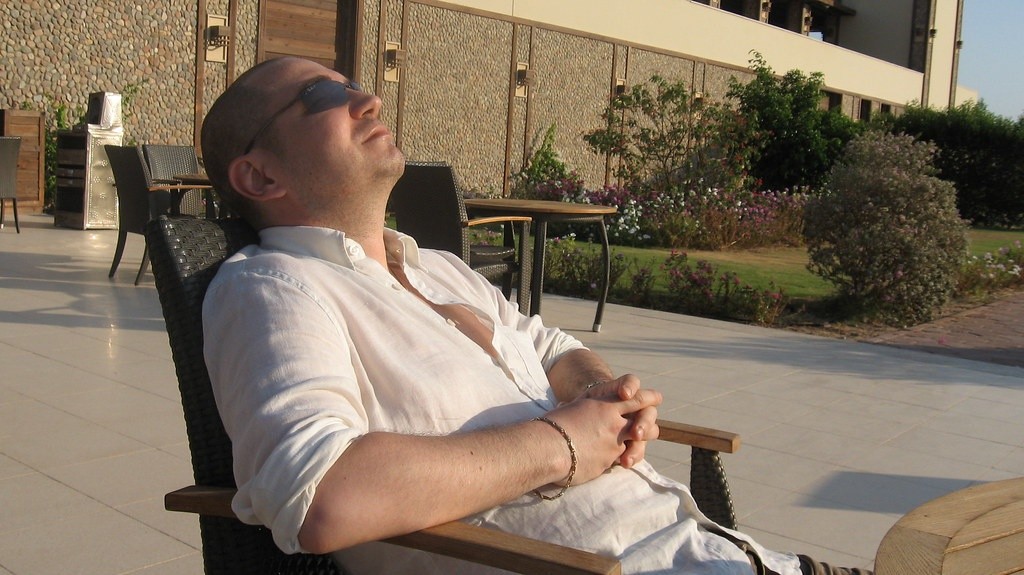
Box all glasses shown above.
[241,79,364,155]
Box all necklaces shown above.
[387,264,409,290]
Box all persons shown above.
[200,57,877,574]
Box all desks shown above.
[465,199,619,333]
[173,174,217,219]
[876,477,1024,575]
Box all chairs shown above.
[143,144,202,217]
[103,145,215,287]
[141,213,741,575]
[391,160,534,313]
[0,135,23,233]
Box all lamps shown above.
[762,2,772,12]
[211,25,231,47]
[518,69,535,86]
[616,85,628,95]
[805,17,813,26]
[695,98,704,110]
[957,41,963,49]
[387,49,406,68]
[930,29,937,38]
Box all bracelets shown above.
[529,415,577,501]
[586,379,609,389]
